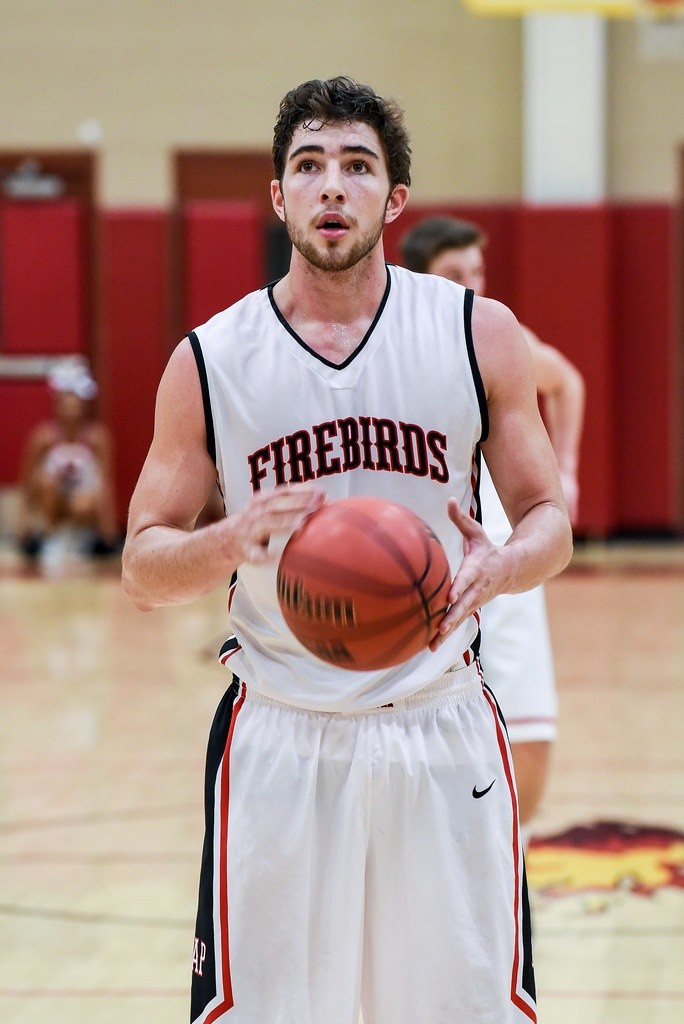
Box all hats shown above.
[48,360,95,398]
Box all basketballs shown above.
[276,495,453,672]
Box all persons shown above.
[404,211,585,858]
[116,80,575,1023]
[17,357,127,567]
